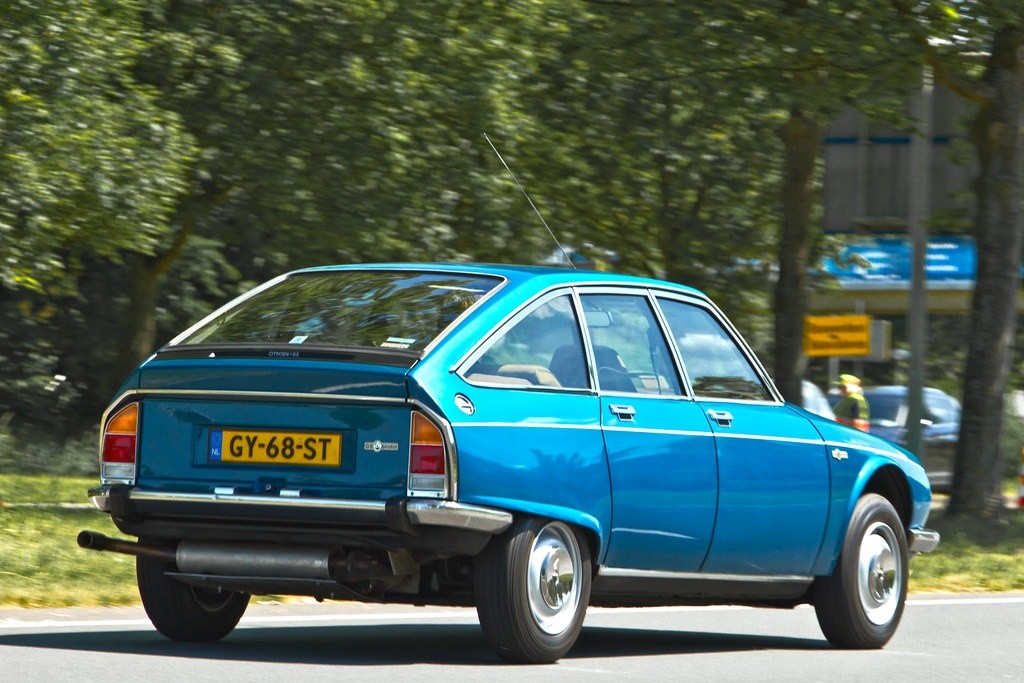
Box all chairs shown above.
[549,344,638,392]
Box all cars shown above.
[770,375,838,422]
[74,261,940,664]
[827,384,962,492]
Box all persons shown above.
[835,373,870,433]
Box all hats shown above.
[831,374,861,385]
